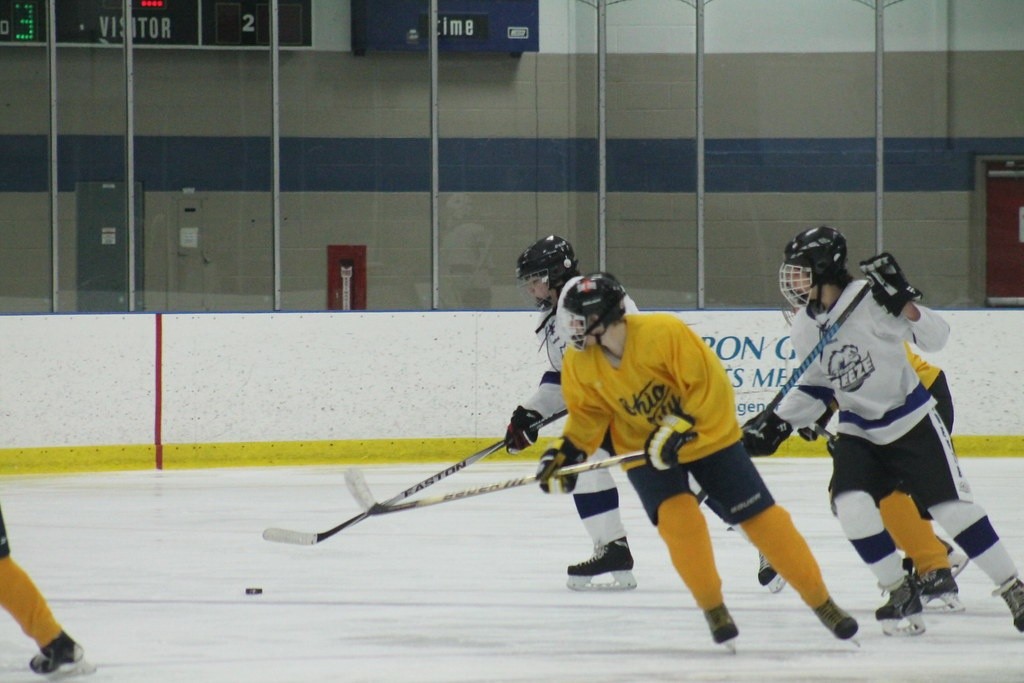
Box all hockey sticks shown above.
[695,280,871,504]
[263,406,700,547]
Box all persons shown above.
[0,503,85,676]
[439,197,496,308]
[535,272,859,645]
[781,297,963,597]
[504,235,777,586]
[739,226,1024,633]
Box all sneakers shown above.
[703,604,739,655]
[874,557,926,637]
[935,535,969,578]
[566,536,637,591]
[813,596,860,648]
[757,551,785,594]
[992,575,1024,632]
[915,567,966,615]
[29,631,97,680]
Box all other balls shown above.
[245,588,263,595]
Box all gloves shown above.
[740,410,794,457]
[535,436,587,494]
[797,406,833,441]
[644,410,695,473]
[505,405,544,454]
[859,252,923,318]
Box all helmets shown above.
[779,225,847,307]
[554,272,627,352]
[516,235,579,308]
